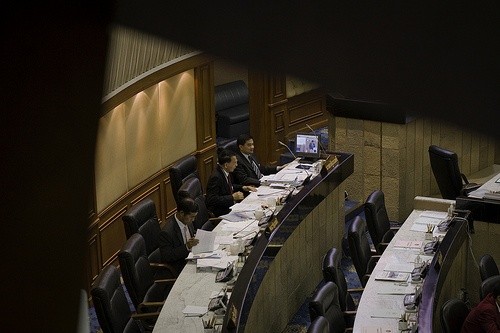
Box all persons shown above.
[460,283,500,333]
[309,140,316,152]
[206,149,257,217]
[230,134,290,188]
[159,198,200,272]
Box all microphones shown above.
[306,124,328,158]
[277,141,312,180]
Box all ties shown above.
[183,226,190,242]
[248,156,261,180]
[227,176,232,193]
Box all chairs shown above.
[92,136,500,333]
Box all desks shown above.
[456,174,500,303]
[353,196,474,333]
[152,151,354,333]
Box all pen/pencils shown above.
[401,311,411,322]
[412,323,416,332]
[427,224,435,233]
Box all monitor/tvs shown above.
[295,133,320,163]
